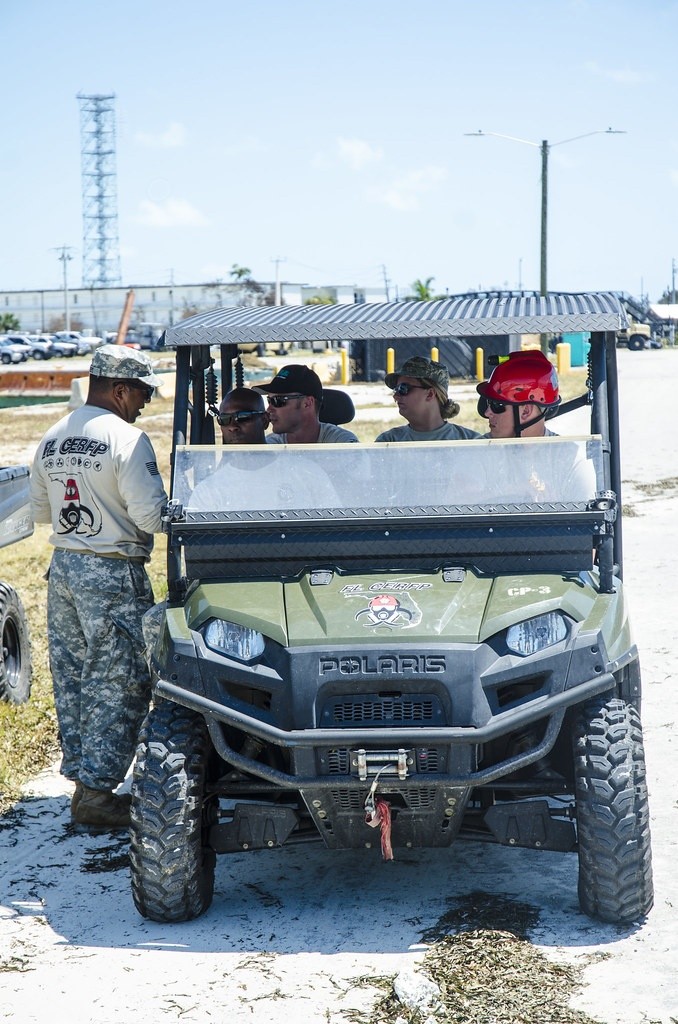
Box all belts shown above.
[57,545,143,563]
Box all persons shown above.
[250,364,362,444]
[374,356,483,442]
[469,350,596,503]
[187,387,342,512]
[28,345,169,831]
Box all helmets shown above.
[476,354,562,407]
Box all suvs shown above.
[130,291,653,924]
[615,313,651,351]
[0,465,34,703]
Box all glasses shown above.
[394,384,427,396]
[113,381,154,398]
[267,395,307,408]
[487,399,511,414]
[217,410,265,426]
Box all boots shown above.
[70,781,133,831]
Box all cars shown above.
[0,331,103,364]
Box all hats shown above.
[251,364,323,400]
[384,356,449,390]
[89,345,164,387]
